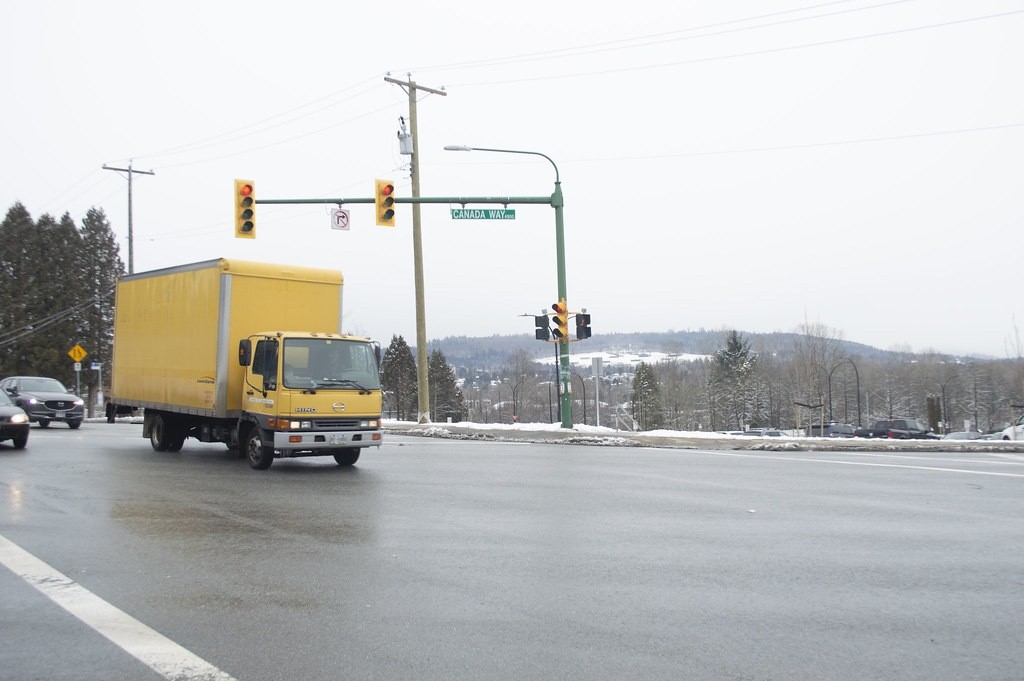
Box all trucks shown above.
[110,258,383,473]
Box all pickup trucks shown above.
[855,418,940,439]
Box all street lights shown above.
[442,146,575,430]
[922,374,960,434]
[810,362,849,423]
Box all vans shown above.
[806,424,854,438]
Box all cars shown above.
[0,387,29,449]
[1001,414,1024,440]
[0,377,85,428]
[943,432,984,441]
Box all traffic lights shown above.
[575,314,592,341]
[377,180,397,227]
[551,303,568,339]
[235,179,259,238]
[534,314,551,341]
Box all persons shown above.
[320,349,353,379]
